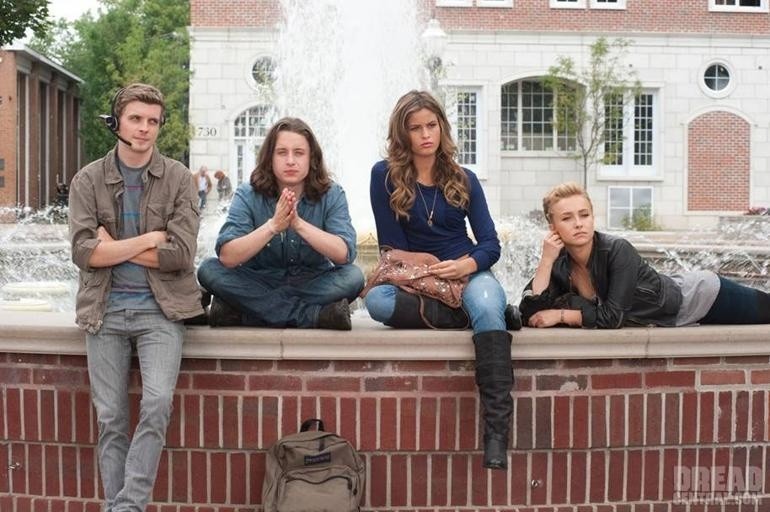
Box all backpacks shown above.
[260,420,366,512]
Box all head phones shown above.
[97,86,166,132]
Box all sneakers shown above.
[318,297,352,330]
[209,294,240,327]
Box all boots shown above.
[471,329,514,470]
[384,291,522,330]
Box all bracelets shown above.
[267,219,278,235]
[560,307,565,323]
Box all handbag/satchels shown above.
[359,244,469,330]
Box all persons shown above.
[197,117,365,330]
[193,165,211,210]
[215,171,232,211]
[521,185,770,329]
[68,84,204,512]
[364,90,521,470]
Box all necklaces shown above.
[412,180,440,226]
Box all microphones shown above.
[109,127,132,146]
